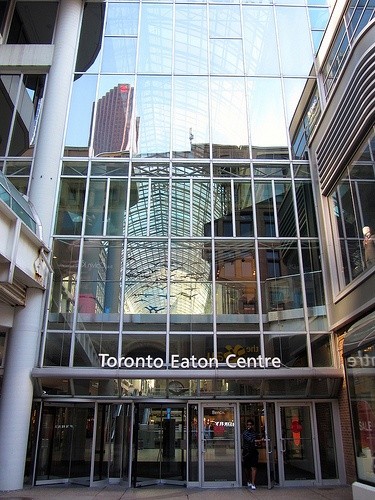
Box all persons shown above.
[241,418,265,490]
[242,290,247,311]
[249,296,256,310]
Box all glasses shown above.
[247,424,252,426]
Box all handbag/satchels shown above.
[247,481,256,489]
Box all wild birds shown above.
[122,255,209,315]
